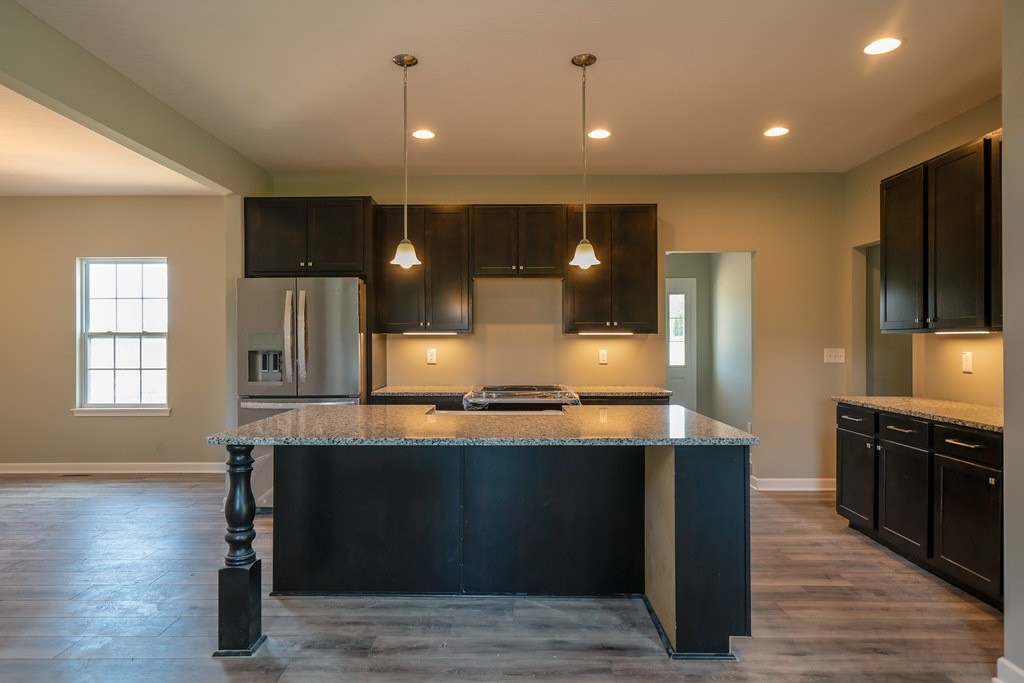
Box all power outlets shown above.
[598,350,608,364]
[963,351,972,373]
[427,347,436,364]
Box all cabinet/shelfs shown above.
[373,203,476,333]
[932,422,1005,612]
[878,136,990,333]
[560,202,660,335]
[470,202,565,280]
[244,195,375,279]
[835,405,932,564]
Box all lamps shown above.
[570,50,601,272]
[388,53,423,270]
